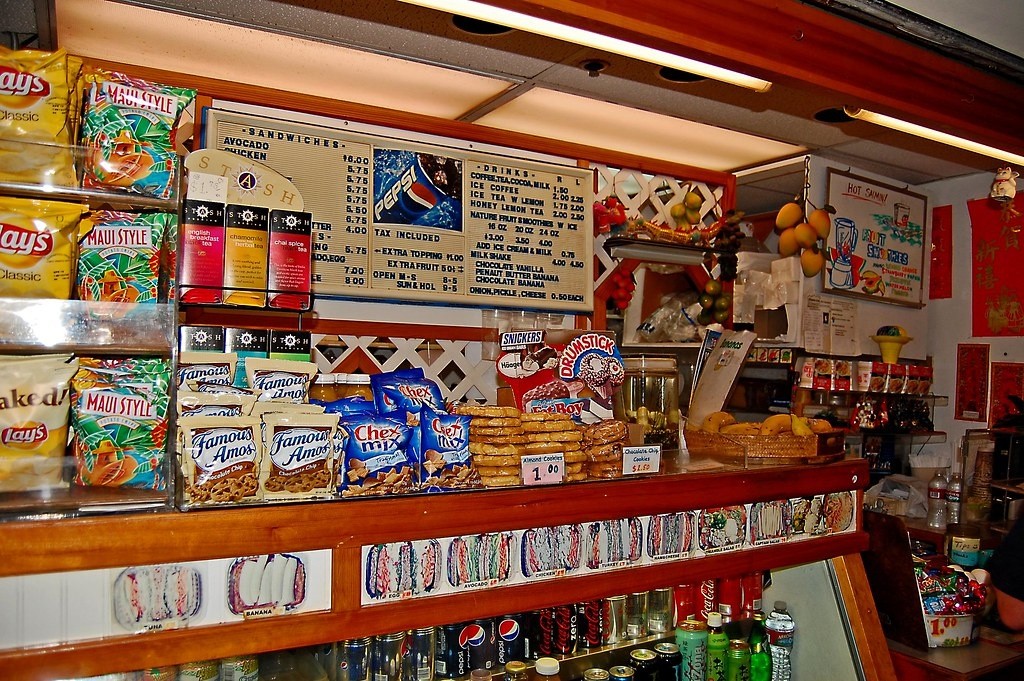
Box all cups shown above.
[972,447,993,505]
[481,309,566,361]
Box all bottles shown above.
[765,600,795,681]
[309,372,373,402]
[706,612,730,681]
[926,469,948,530]
[946,472,963,523]
[747,611,772,681]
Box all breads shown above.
[457,407,628,486]
[697,411,835,435]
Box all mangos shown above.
[696,279,729,326]
[775,202,830,278]
[671,192,702,232]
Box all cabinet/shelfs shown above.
[844,393,950,444]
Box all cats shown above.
[990,166,1020,201]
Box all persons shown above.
[990,511,1024,629]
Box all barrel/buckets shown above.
[910,466,950,482]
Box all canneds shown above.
[315,569,762,681]
[136,653,259,681]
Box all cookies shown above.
[341,450,482,495]
[186,469,331,503]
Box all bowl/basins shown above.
[964,504,992,521]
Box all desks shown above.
[894,513,981,559]
